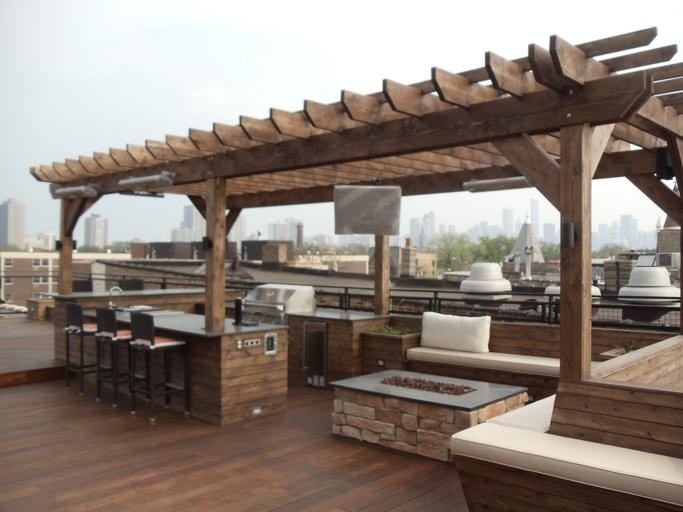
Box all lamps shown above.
[462,175,528,192]
[55,183,100,199]
[115,171,176,191]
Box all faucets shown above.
[107,287,124,310]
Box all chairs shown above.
[93,308,149,408]
[64,302,98,396]
[119,279,143,290]
[72,280,92,292]
[127,311,191,423]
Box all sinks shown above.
[118,305,163,312]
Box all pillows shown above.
[419,311,491,354]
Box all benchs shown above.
[405,345,607,401]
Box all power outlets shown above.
[264,333,277,355]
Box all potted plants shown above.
[599,341,642,363]
[360,324,421,371]
